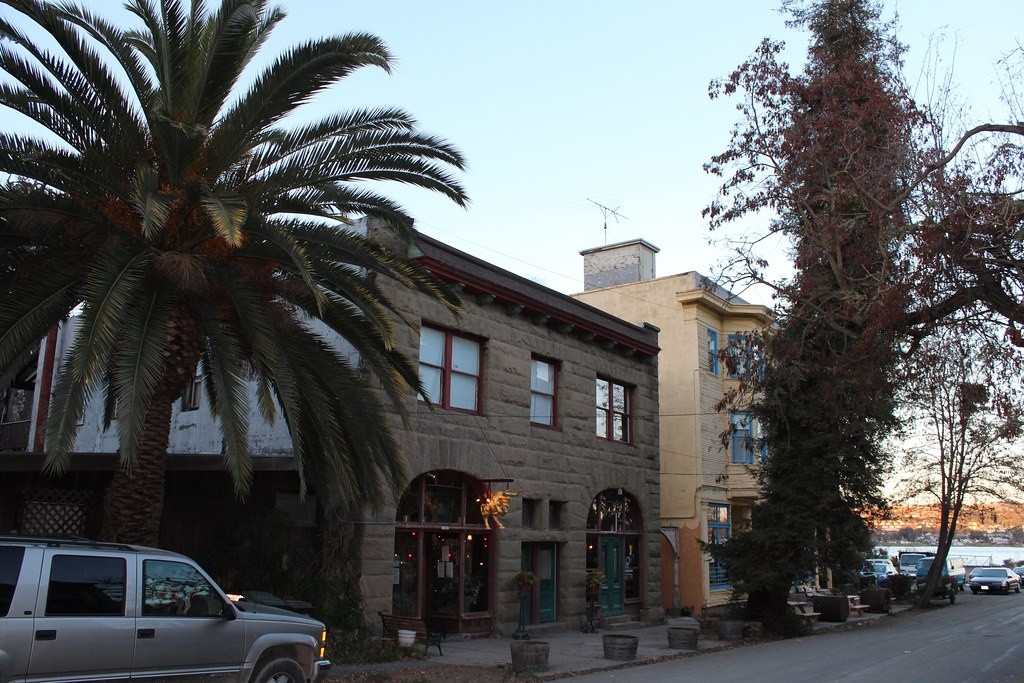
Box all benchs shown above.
[852,605,870,611]
[377,610,444,658]
[799,613,821,619]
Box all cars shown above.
[865,547,1024,601]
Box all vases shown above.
[602,634,639,660]
[667,627,699,650]
[510,641,550,672]
[398,631,417,646]
[717,620,743,641]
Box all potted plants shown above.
[512,572,537,640]
[582,570,605,633]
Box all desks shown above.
[847,596,865,618]
[788,602,812,624]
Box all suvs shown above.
[0,534,335,683]
